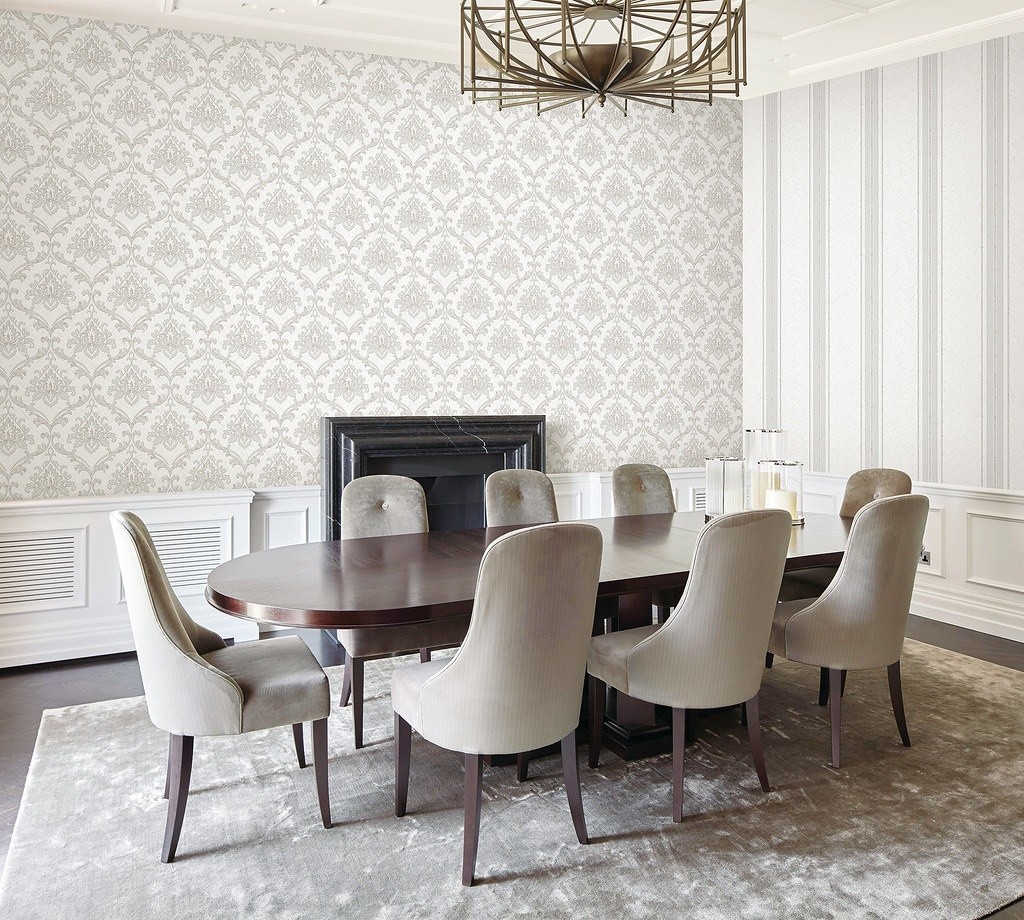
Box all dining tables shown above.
[201,505,903,791]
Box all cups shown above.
[705,428,805,526]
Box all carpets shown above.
[0,610,1024,920]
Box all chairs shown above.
[387,523,606,885]
[762,467,914,705]
[762,491,932,768]
[338,472,439,748]
[614,462,678,517]
[107,508,335,863]
[583,507,796,824]
[485,468,559,530]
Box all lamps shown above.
[457,0,748,120]
